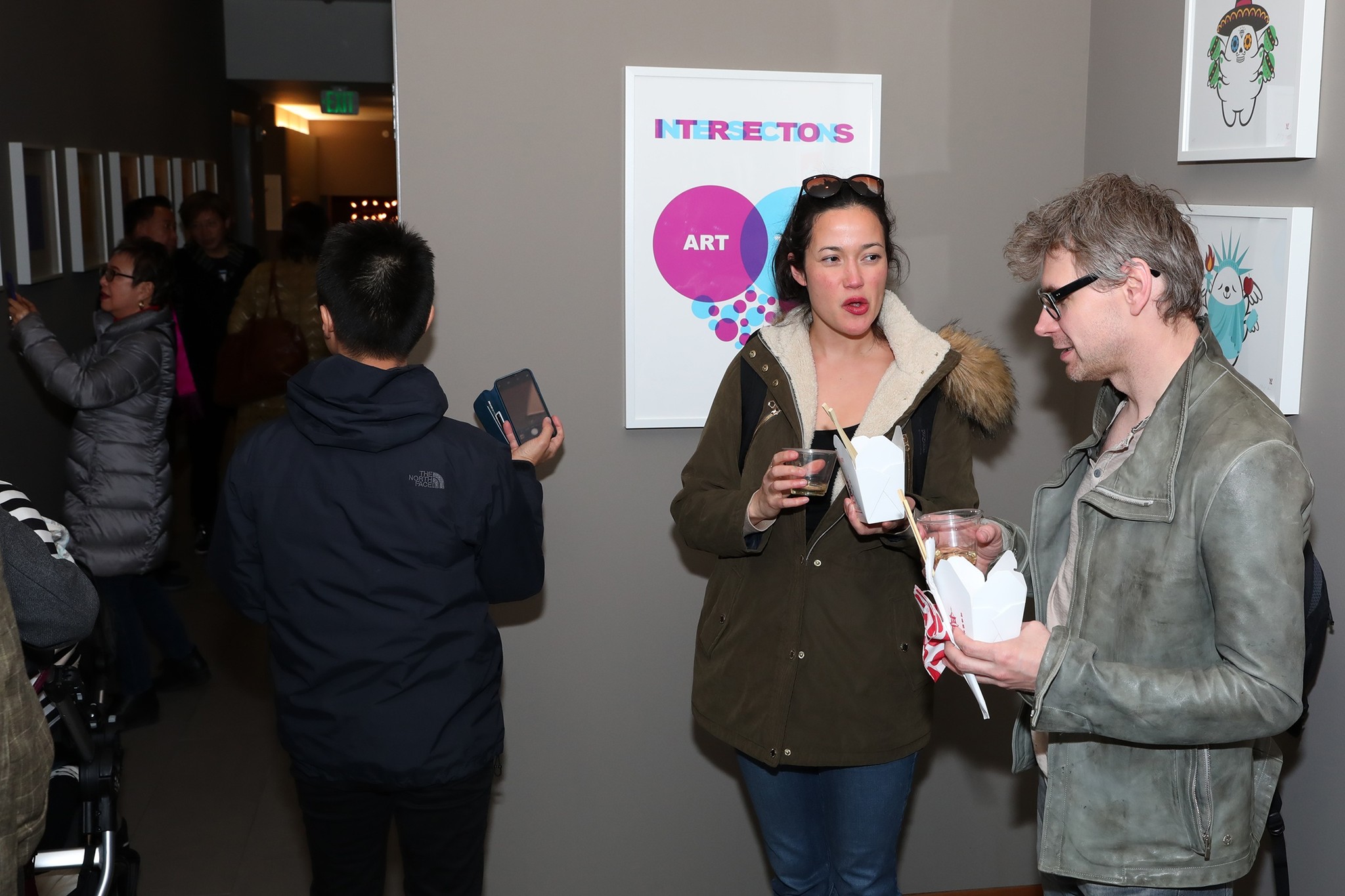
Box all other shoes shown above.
[152,648,210,691]
[159,571,192,592]
[109,690,158,728]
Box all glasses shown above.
[1036,263,1161,321]
[98,266,138,283]
[790,174,885,235]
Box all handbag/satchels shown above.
[1284,539,1334,752]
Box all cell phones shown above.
[5,271,17,300]
[496,368,556,446]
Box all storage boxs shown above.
[834,425,904,524]
[924,535,1028,646]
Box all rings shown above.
[9,316,12,321]
[770,462,773,468]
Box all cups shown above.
[781,447,838,497]
[917,508,982,566]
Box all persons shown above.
[0,476,102,896]
[205,219,564,896]
[9,191,334,734]
[915,174,1314,896]
[670,173,1014,896]
